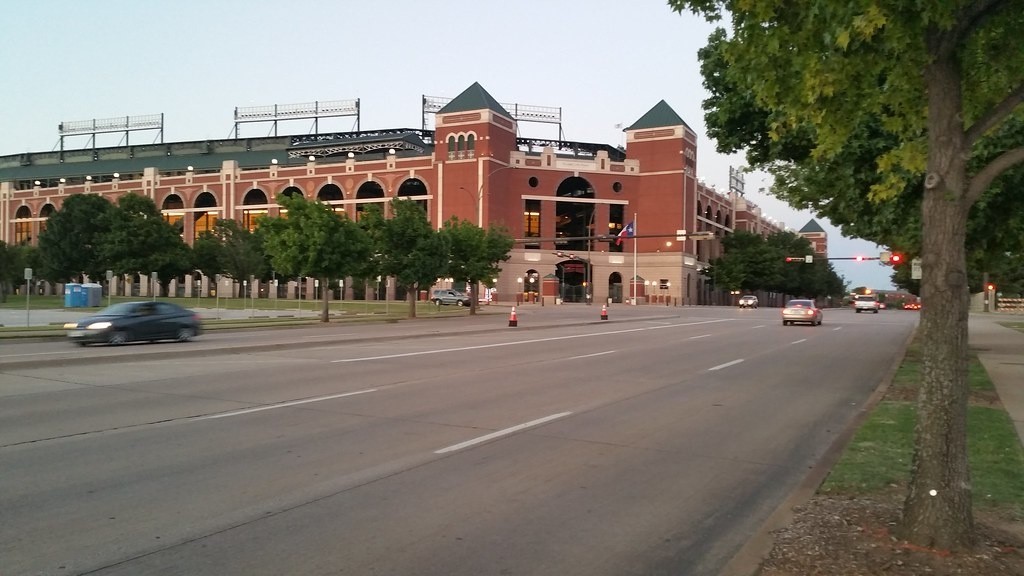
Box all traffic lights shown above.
[557,251,563,257]
[891,255,901,262]
[570,254,574,259]
[786,258,804,262]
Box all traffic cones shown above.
[509,306,517,320]
[600,304,607,315]
[486,299,489,305]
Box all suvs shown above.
[431,290,471,307]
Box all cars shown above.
[739,295,758,309]
[67,301,203,347]
[854,294,886,314]
[782,300,823,326]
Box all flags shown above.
[616,222,634,245]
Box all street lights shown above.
[588,203,606,260]
[461,166,517,225]
